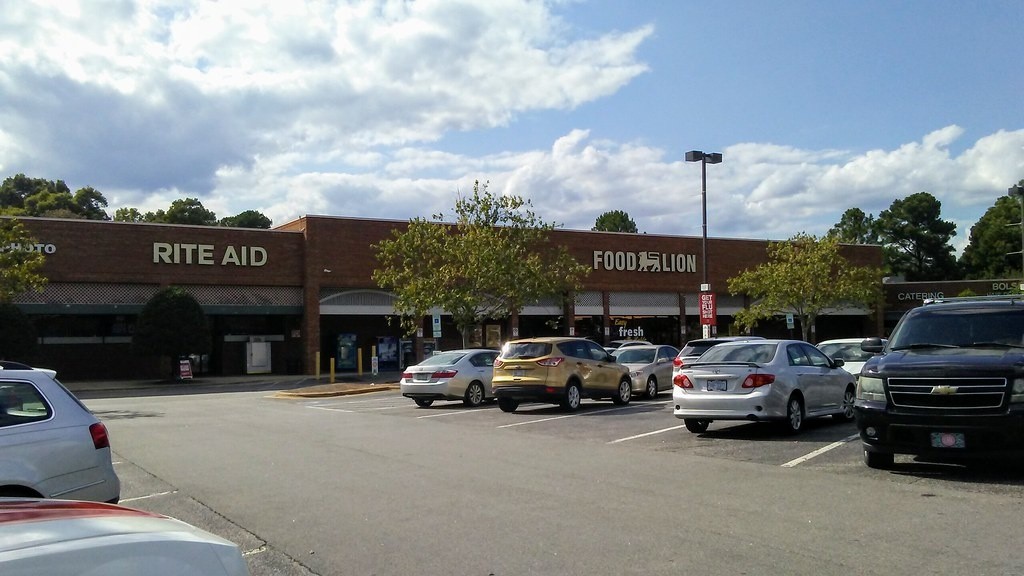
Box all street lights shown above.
[685,149,724,339]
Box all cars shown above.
[672,340,858,435]
[808,337,891,387]
[602,340,654,356]
[0,497,250,576]
[609,345,681,399]
[400,348,499,408]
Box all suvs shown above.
[0,360,121,508]
[856,293,1024,471]
[672,336,771,391]
[492,336,633,413]
